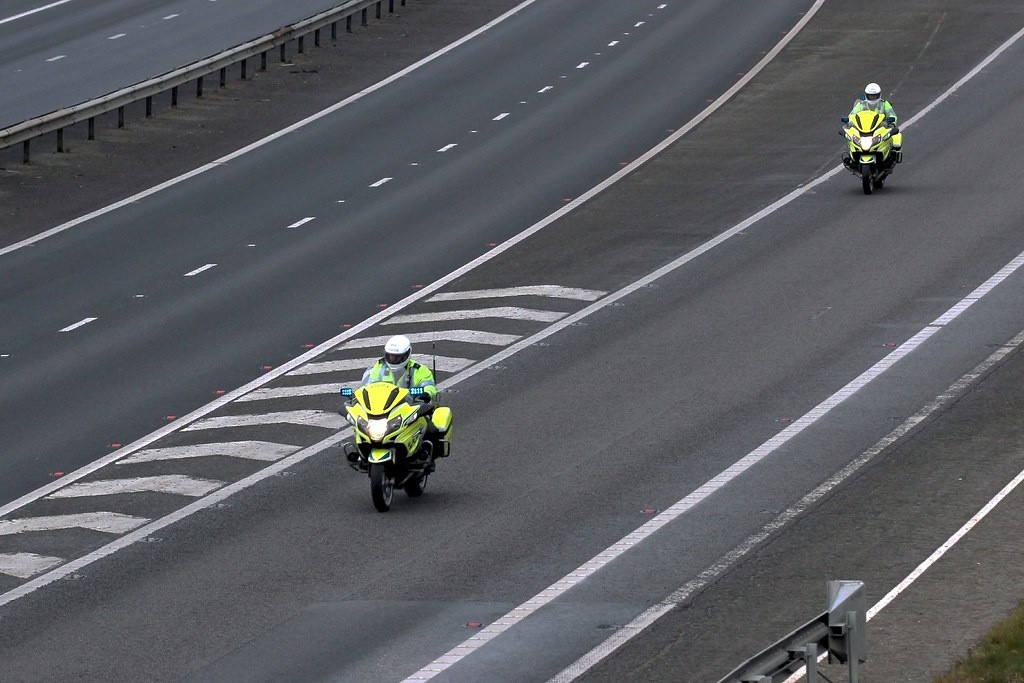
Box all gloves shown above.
[422,392,431,403]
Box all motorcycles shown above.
[838,92,903,195]
[338,341,454,513]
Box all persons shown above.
[845,82,897,161]
[353,334,439,473]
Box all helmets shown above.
[865,83,882,106]
[384,335,412,372]
